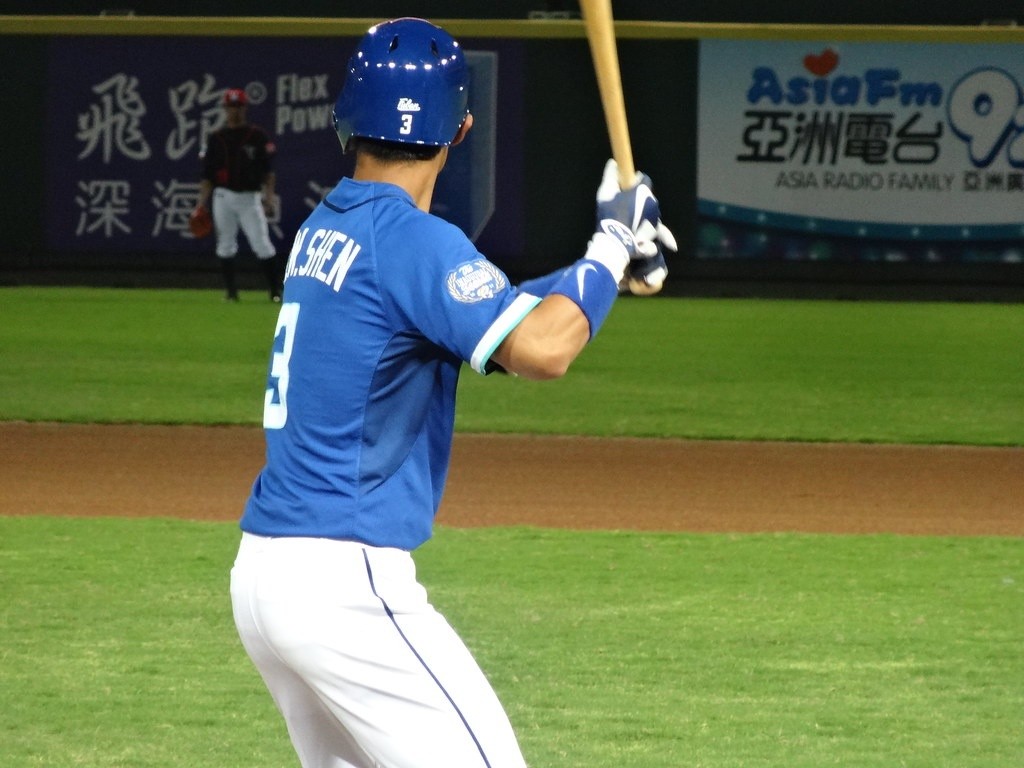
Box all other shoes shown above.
[271,288,281,302]
[227,289,238,302]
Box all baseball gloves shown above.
[189,205,214,238]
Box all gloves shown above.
[584,158,678,296]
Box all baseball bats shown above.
[579,0,663,295]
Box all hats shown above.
[223,89,247,107]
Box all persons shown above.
[227,15,680,768]
[189,88,283,304]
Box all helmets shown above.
[332,18,468,145]
